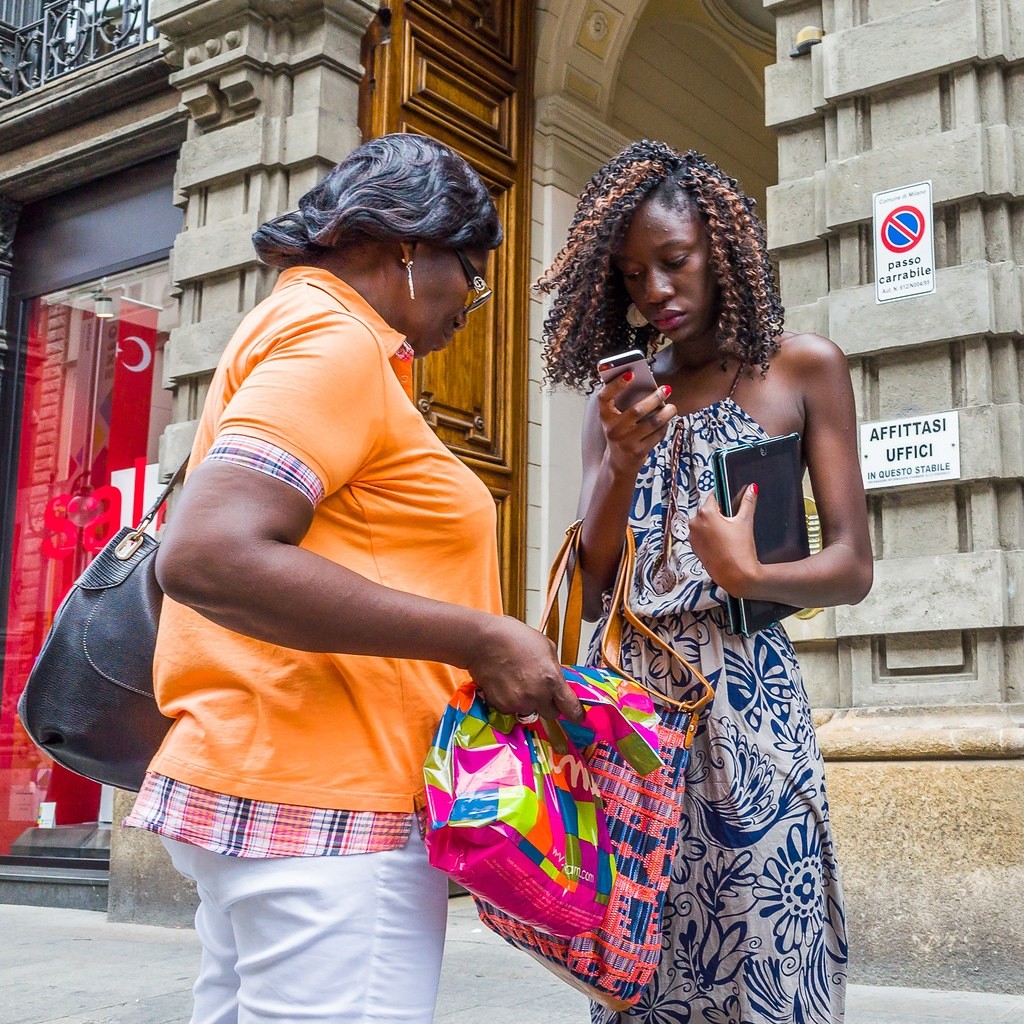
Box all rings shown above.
[516,712,540,724]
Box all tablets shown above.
[719,431,811,638]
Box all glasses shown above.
[445,243,493,315]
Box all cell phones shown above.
[597,350,666,424]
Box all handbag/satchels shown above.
[424,663,665,937]
[16,452,174,793]
[470,515,715,1012]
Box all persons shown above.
[533,141,874,1024]
[121,138,585,1024]
